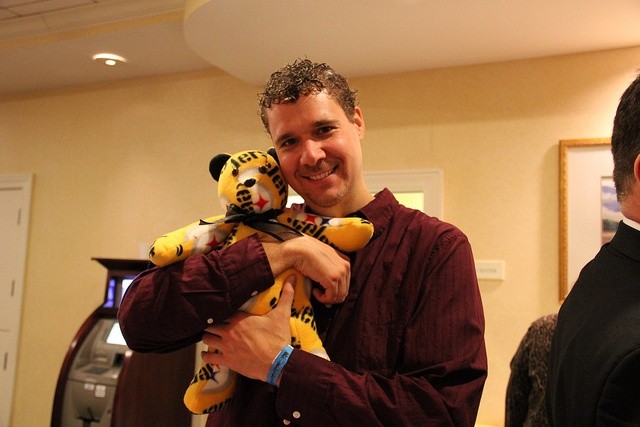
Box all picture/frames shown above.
[558,139,624,304]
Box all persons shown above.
[544,75,639,426]
[115,58,487,427]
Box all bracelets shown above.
[266,344,295,385]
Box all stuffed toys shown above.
[148,145,376,417]
